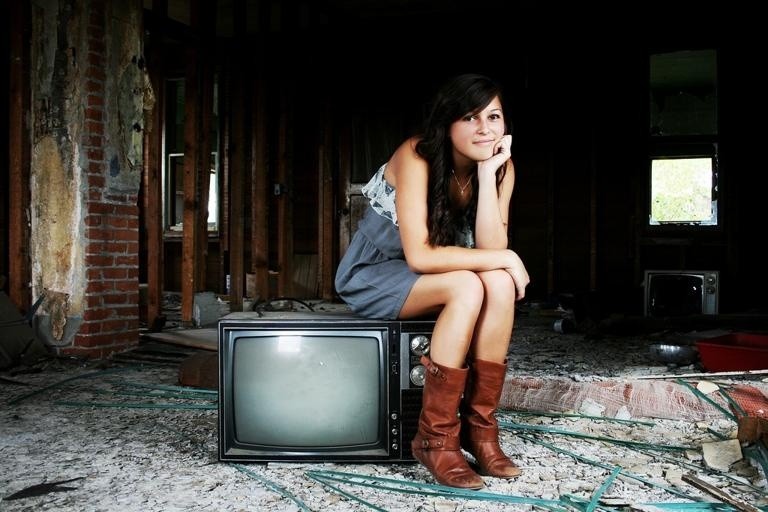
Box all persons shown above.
[335,70,533,490]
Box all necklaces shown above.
[452,170,473,197]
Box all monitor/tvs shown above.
[642,49,722,319]
[216,310,437,465]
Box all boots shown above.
[460,356,522,479]
[411,353,485,490]
